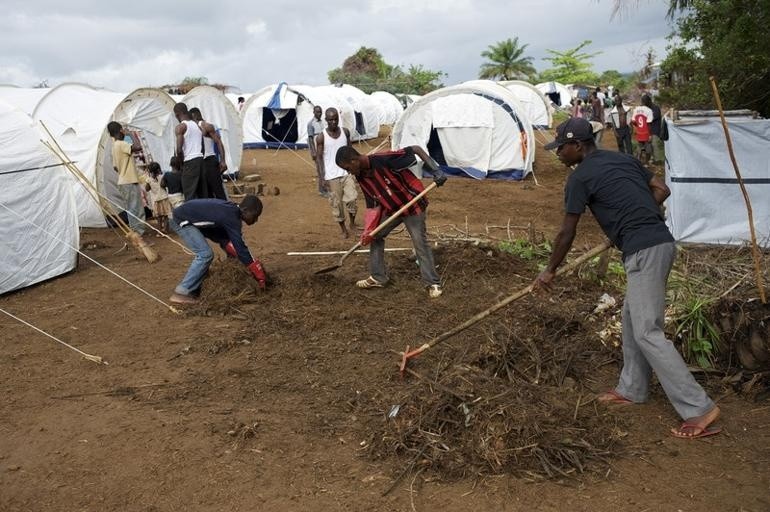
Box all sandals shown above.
[427,282,445,299]
[355,276,389,292]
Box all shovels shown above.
[312,181,437,274]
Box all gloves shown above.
[222,238,237,257]
[248,259,272,288]
[421,156,447,188]
[360,210,381,246]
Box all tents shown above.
[0,109,81,296]
[1,83,180,229]
[168,84,243,181]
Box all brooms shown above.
[37,118,163,265]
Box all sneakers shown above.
[169,292,203,305]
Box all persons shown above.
[107,121,145,237]
[173,102,209,200]
[569,85,662,166]
[145,157,185,239]
[190,107,228,200]
[315,105,363,239]
[167,194,267,304]
[335,145,448,299]
[307,106,329,199]
[530,117,723,440]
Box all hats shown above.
[542,118,595,152]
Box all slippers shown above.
[667,420,722,438]
[596,389,639,408]
